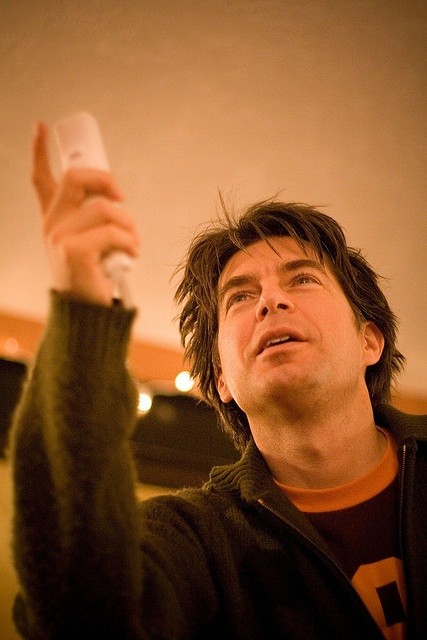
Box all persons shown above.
[13,120,426,640]
